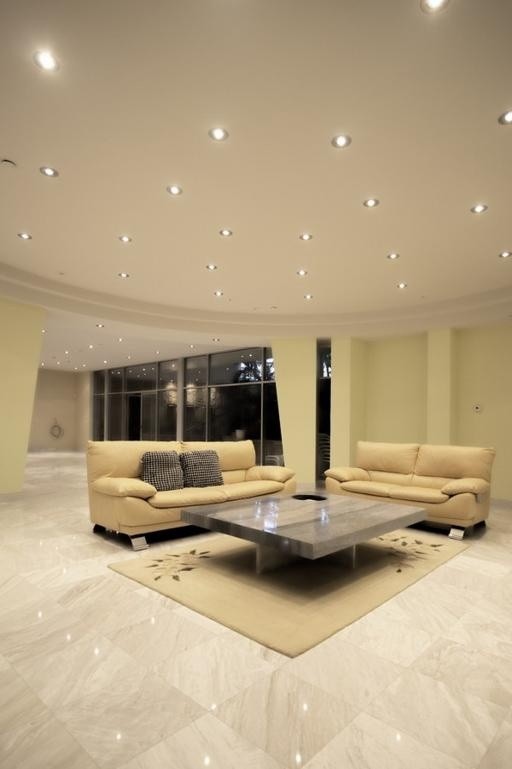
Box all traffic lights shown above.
[139,450,186,493]
[177,450,226,488]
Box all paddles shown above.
[106,527,472,660]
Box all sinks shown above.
[84,438,298,553]
[322,440,497,541]
